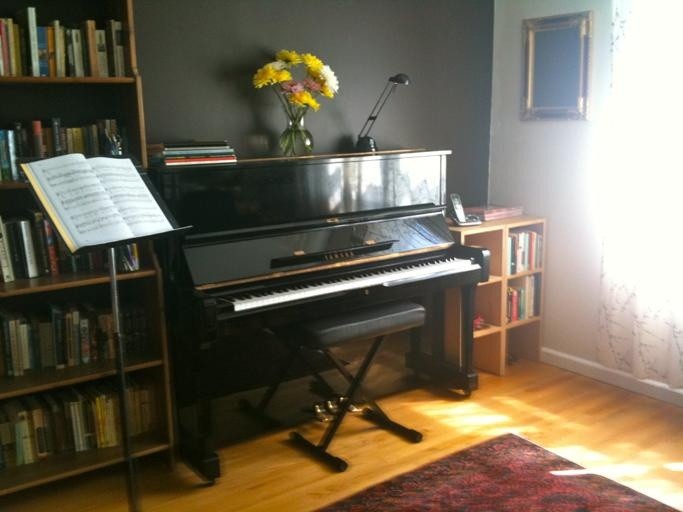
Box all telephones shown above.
[449,192,480,226]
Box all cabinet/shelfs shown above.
[446,209,545,374]
[0,0,173,500]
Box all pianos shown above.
[148,147,490,483]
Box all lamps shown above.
[354,72,413,152]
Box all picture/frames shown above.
[522,8,592,125]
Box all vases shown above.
[273,102,314,155]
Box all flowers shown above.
[249,47,341,115]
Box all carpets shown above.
[306,432,681,512]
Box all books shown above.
[1,299,148,377]
[0,119,119,182]
[1,207,140,284]
[0,7,126,78]
[509,227,538,322]
[145,140,238,169]
[465,204,523,221]
[1,383,154,469]
[20,154,177,257]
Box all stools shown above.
[238,310,351,440]
[287,300,431,471]
